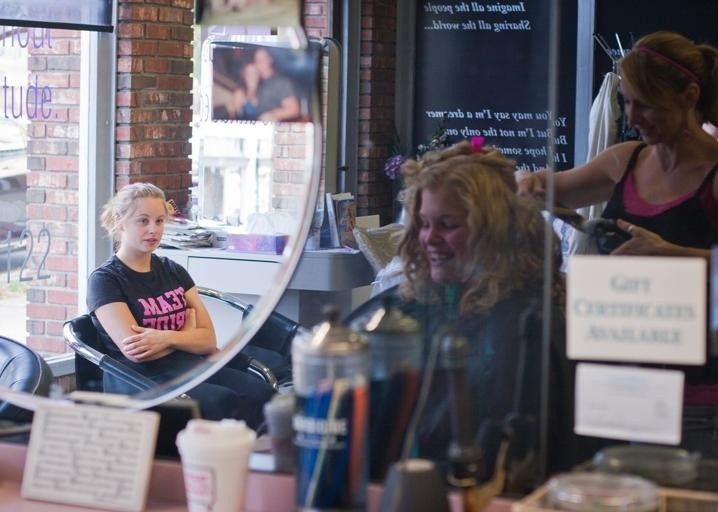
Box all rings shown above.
[626,224,635,232]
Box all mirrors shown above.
[1,0,717,511]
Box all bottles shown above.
[352,295,424,484]
[290,305,370,512]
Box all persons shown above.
[223,48,300,123]
[516,29,718,257]
[85,183,270,438]
[366,141,574,502]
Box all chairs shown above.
[0,335,54,449]
[60,284,311,463]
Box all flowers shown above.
[381,125,452,184]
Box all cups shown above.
[549,444,702,512]
[381,456,452,512]
[264,395,296,472]
[176,418,256,512]
